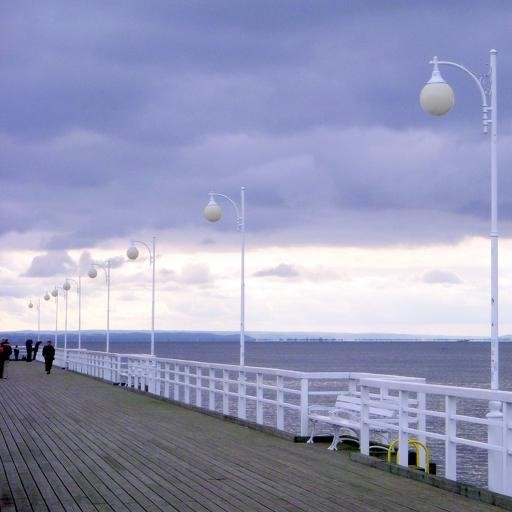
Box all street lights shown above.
[420,46,506,496]
[44,286,58,365]
[127,235,157,392]
[28,298,40,361]
[204,186,247,419]
[51,280,67,368]
[63,274,82,372]
[88,259,112,381]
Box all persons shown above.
[0,336,42,379]
[42,340,55,374]
